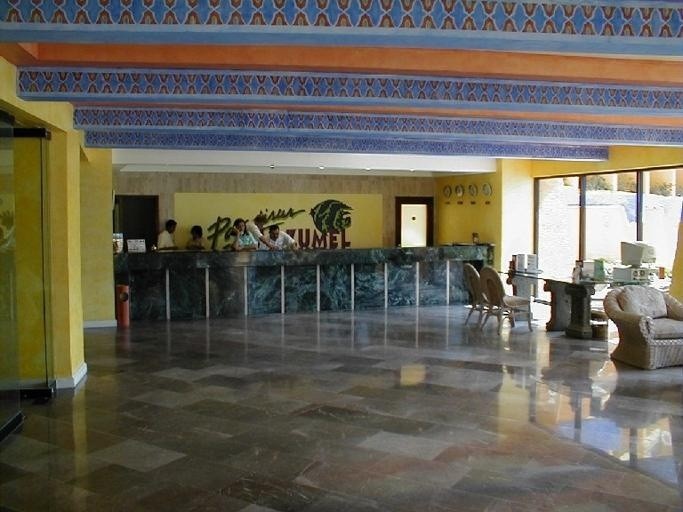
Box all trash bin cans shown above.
[116,283,130,329]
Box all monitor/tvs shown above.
[620,241,657,268]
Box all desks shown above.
[498,267,609,340]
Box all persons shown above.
[231,218,255,251]
[184,226,208,251]
[156,219,177,250]
[259,224,297,250]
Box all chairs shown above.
[603,283,683,371]
[462,262,533,334]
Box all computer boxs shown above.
[613,266,657,284]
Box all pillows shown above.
[617,285,667,320]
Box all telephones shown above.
[233,225,243,234]
